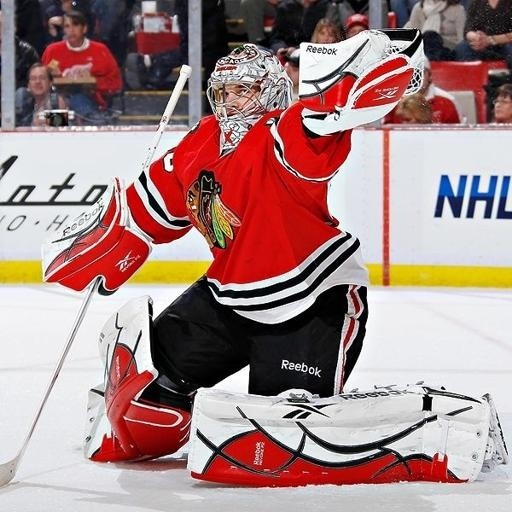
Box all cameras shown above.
[45,110,68,126]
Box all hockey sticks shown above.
[2,64,192,485]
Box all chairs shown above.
[14,0,512,127]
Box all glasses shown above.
[493,99,512,105]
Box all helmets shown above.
[206,42,293,159]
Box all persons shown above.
[41,30,508,484]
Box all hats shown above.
[346,13,369,28]
